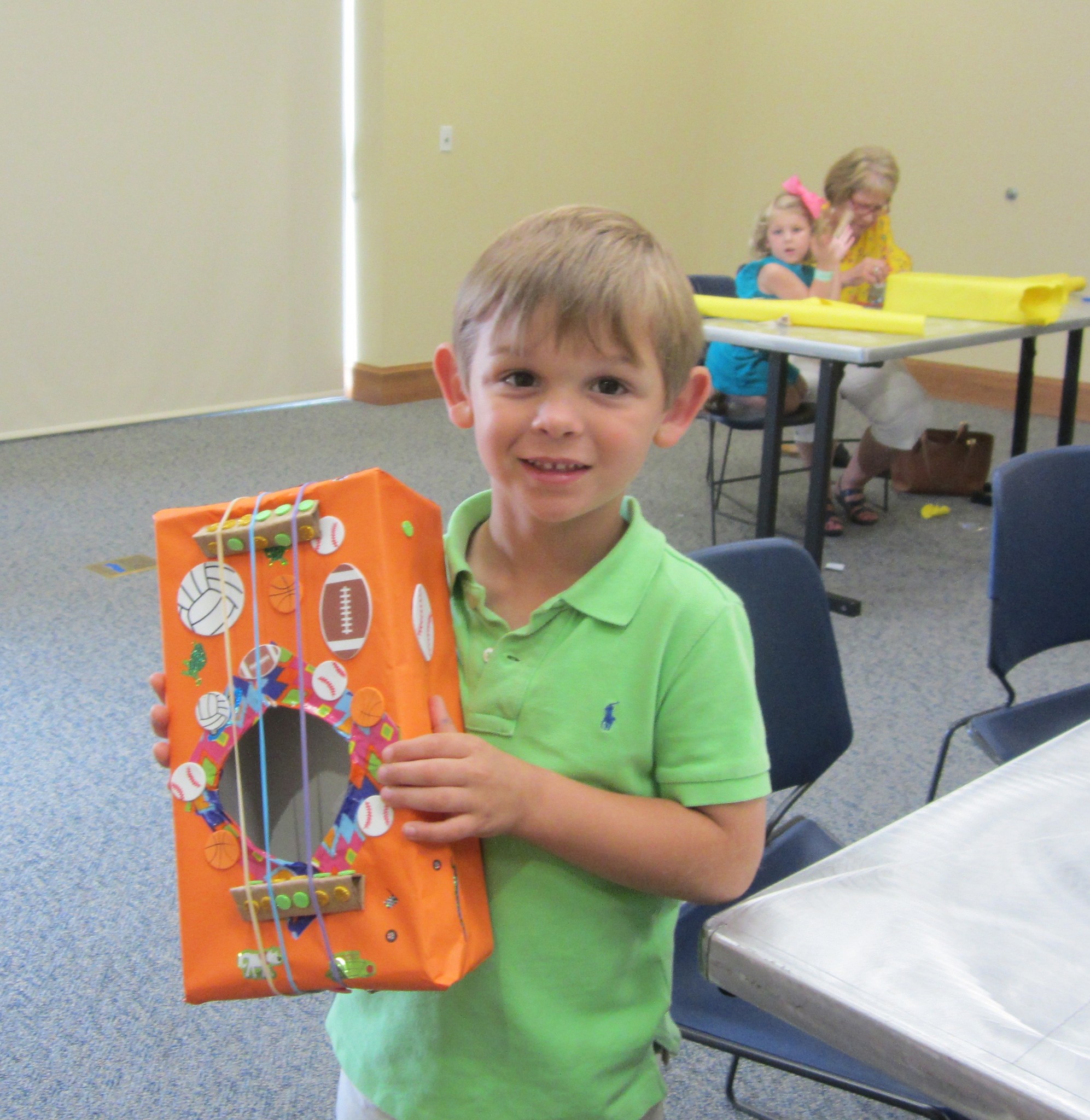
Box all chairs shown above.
[672,534,971,1120]
[689,274,893,537]
[927,445,1090,803]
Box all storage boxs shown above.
[907,430,997,493]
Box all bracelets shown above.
[813,269,833,281]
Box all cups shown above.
[867,265,886,309]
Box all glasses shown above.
[849,195,890,218]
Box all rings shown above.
[873,267,878,274]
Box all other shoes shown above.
[704,392,724,415]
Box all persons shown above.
[706,179,854,423]
[153,205,772,1119]
[784,145,933,534]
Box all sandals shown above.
[834,476,875,524]
[823,502,842,537]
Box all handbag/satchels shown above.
[890,426,993,493]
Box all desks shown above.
[702,299,1089,615]
[702,719,1090,1120]
